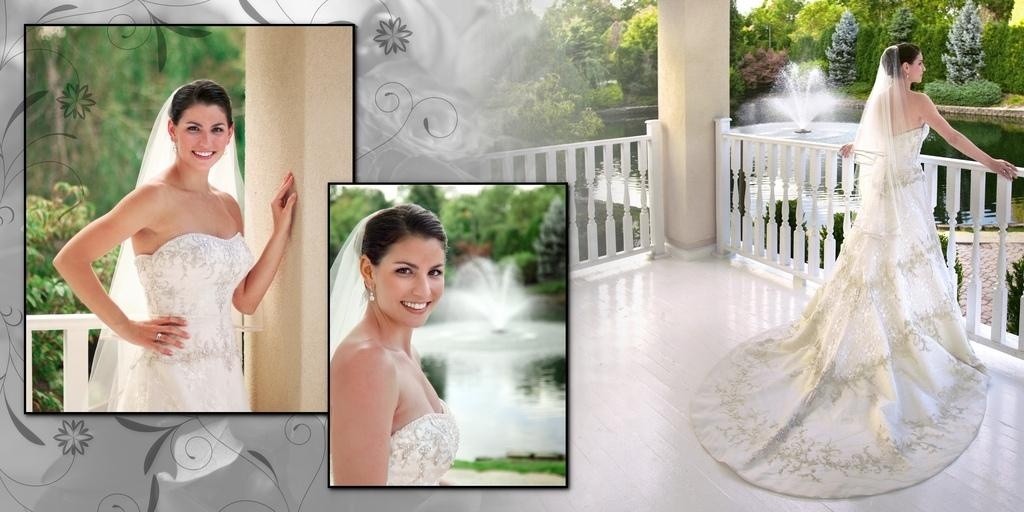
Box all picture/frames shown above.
[22,23,358,417]
[327,181,571,488]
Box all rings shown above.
[156,334,161,341]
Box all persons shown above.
[693,43,1019,499]
[330,203,459,487]
[52,79,296,412]
